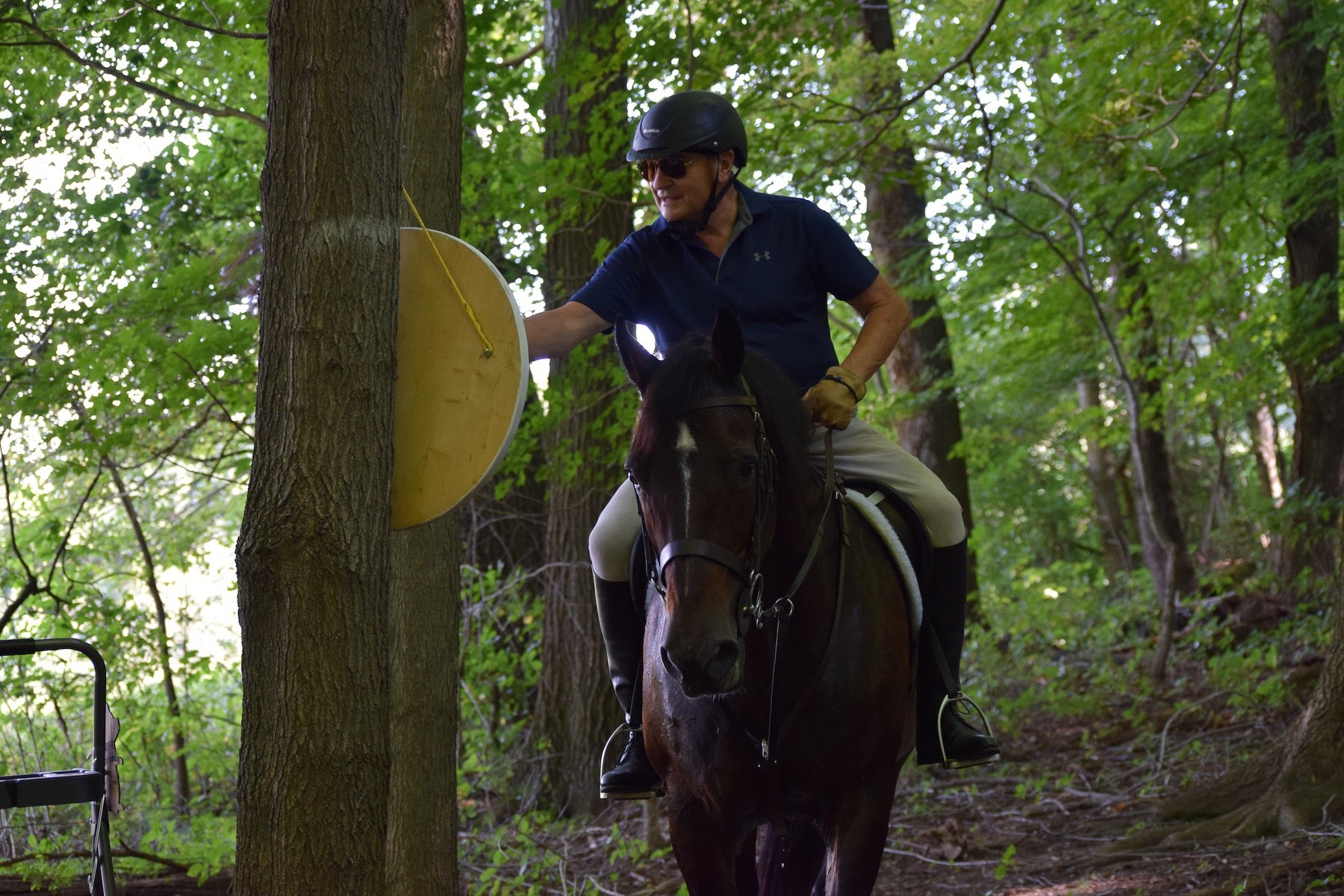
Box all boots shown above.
[590,566,661,794]
[917,525,1000,766]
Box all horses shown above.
[614,321,931,895]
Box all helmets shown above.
[626,90,748,169]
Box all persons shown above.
[502,92,1002,812]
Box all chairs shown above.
[0,638,117,896]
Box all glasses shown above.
[636,151,706,183]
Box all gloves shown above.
[803,364,867,431]
